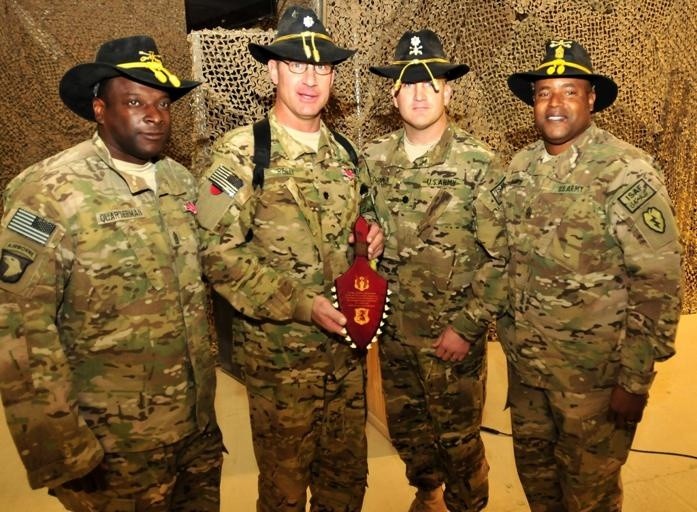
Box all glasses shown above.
[279,59,334,75]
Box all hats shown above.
[368,29,469,96]
[507,40,617,114]
[248,5,355,64]
[59,35,202,122]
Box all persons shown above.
[494,38,682,511]
[0,35,229,512]
[195,6,384,511]
[359,29,506,512]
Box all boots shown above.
[408,486,450,511]
